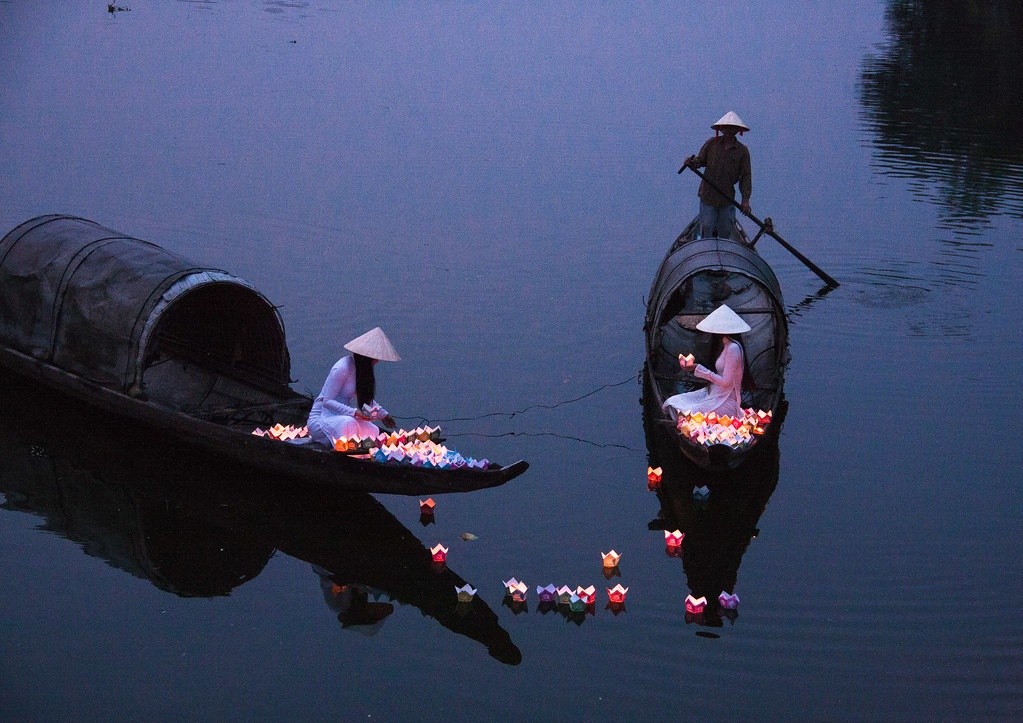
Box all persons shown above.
[307,326,401,446]
[662,304,752,417]
[684,111,753,238]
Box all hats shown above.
[344,327,401,362]
[711,111,750,132]
[696,304,751,334]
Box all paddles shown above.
[679,154,840,290]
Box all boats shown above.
[0,214,530,494]
[643,211,792,479]
